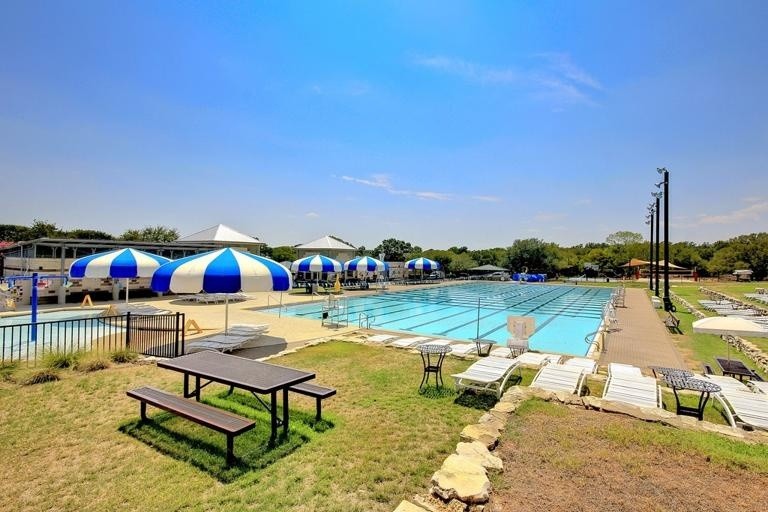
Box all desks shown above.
[156,350,315,441]
[647,365,720,420]
[716,357,755,381]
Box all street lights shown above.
[644,168,671,302]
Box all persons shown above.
[360,274,364,280]
[365,272,370,281]
[372,273,377,280]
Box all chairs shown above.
[368,334,662,411]
[704,374,768,432]
[697,291,768,336]
[183,323,270,356]
[293,274,448,288]
[107,305,172,321]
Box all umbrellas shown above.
[617,257,686,281]
[150,245,293,337]
[68,246,175,312]
[467,263,511,274]
[342,255,389,282]
[290,252,344,284]
[404,256,441,282]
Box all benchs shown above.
[663,311,680,334]
[752,368,765,381]
[126,386,255,466]
[220,382,337,421]
